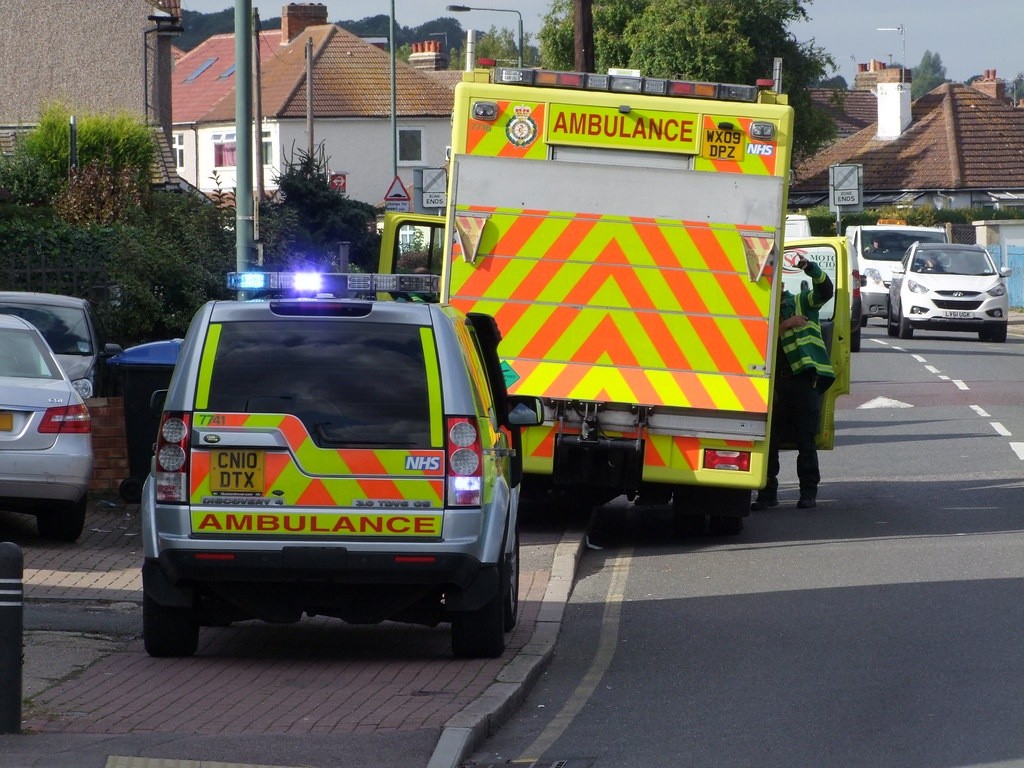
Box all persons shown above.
[396,267,439,303]
[750,252,834,511]
[916,257,944,272]
[864,237,890,253]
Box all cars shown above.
[140,272,544,659]
[887,241,1012,343]
[0,311,94,545]
[0,291,124,403]
[845,240,862,353]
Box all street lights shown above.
[447,5,524,68]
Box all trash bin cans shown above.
[107,337,185,505]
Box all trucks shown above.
[845,226,950,326]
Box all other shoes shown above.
[796,497,817,508]
[752,500,778,510]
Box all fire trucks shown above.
[377,70,853,548]
